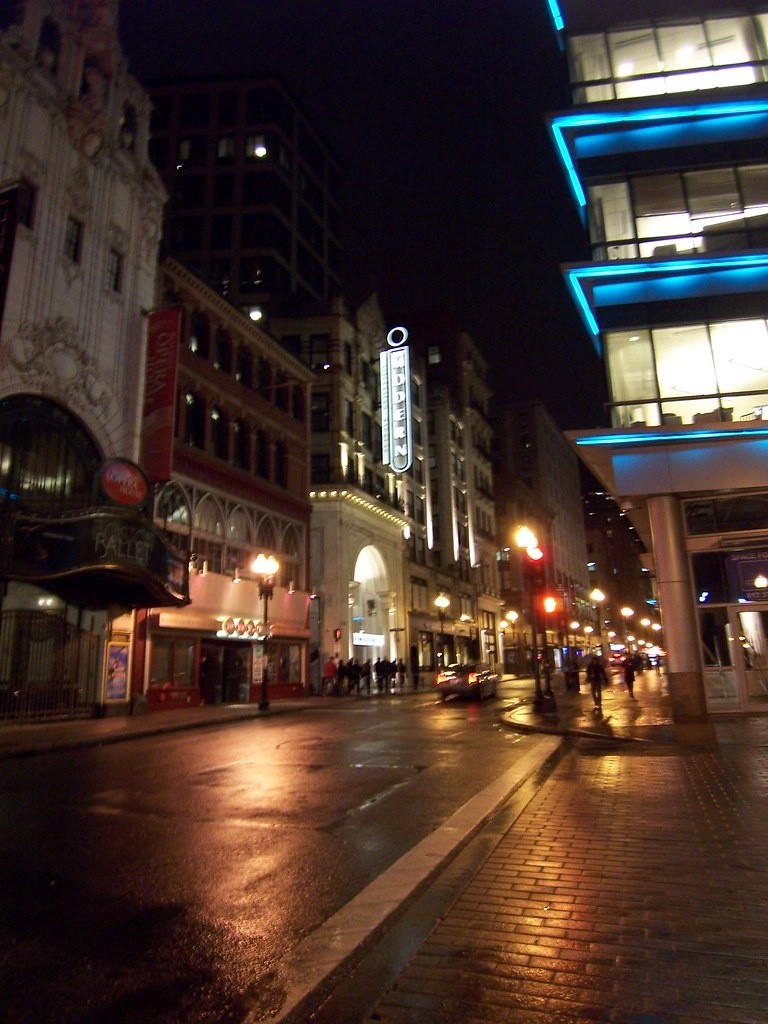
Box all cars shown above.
[437,663,499,704]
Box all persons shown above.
[586,657,609,707]
[321,656,408,696]
[655,655,661,673]
[622,651,643,699]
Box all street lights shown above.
[507,611,520,677]
[590,588,662,657]
[251,553,280,713]
[434,596,450,671]
